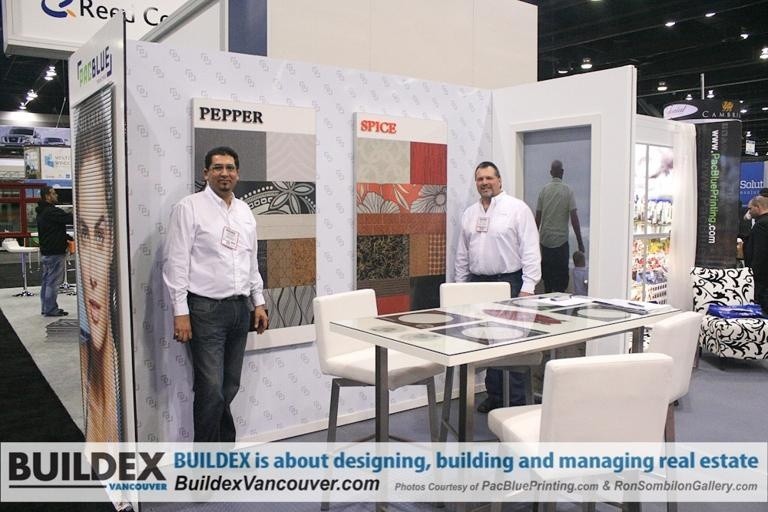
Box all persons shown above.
[36,186,72,318]
[161,147,269,451]
[737,188,768,315]
[532,161,588,296]
[453,162,542,415]
[75,93,128,442]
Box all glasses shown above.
[212,164,235,172]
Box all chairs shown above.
[2,238,41,297]
[439,282,543,509]
[689,265,768,371]
[646,311,704,510]
[311,286,444,511]
[487,351,675,511]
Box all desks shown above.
[29,231,76,289]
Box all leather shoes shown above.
[478,393,505,413]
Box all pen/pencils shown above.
[592,300,612,306]
[628,302,644,308]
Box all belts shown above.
[188,291,247,302]
[472,269,523,279]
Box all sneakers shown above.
[45,309,68,316]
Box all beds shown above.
[329,289,683,509]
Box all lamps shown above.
[557,45,768,137]
[20,64,57,109]
